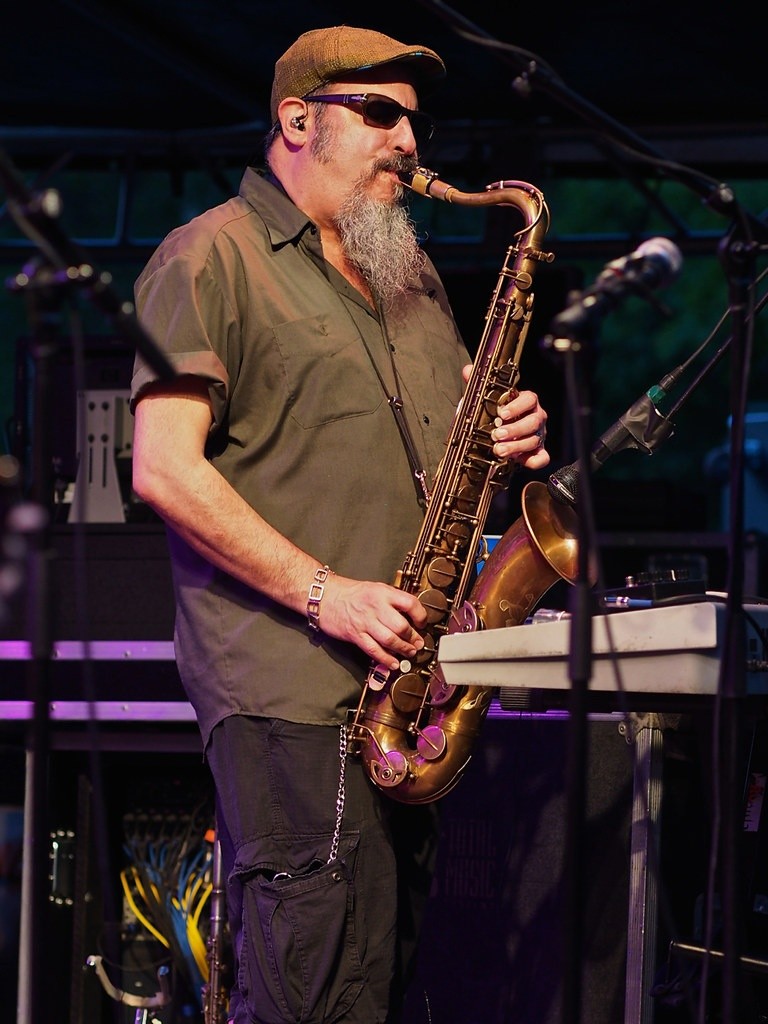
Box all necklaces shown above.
[319,233,433,507]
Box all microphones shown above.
[546,366,684,507]
[552,235,684,341]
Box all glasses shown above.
[265,92,436,151]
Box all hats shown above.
[270,27,447,126]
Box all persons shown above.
[130,22,551,1023]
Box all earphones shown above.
[291,117,305,131]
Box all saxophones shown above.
[335,162,598,804]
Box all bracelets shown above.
[305,564,336,637]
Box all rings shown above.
[537,432,546,446]
